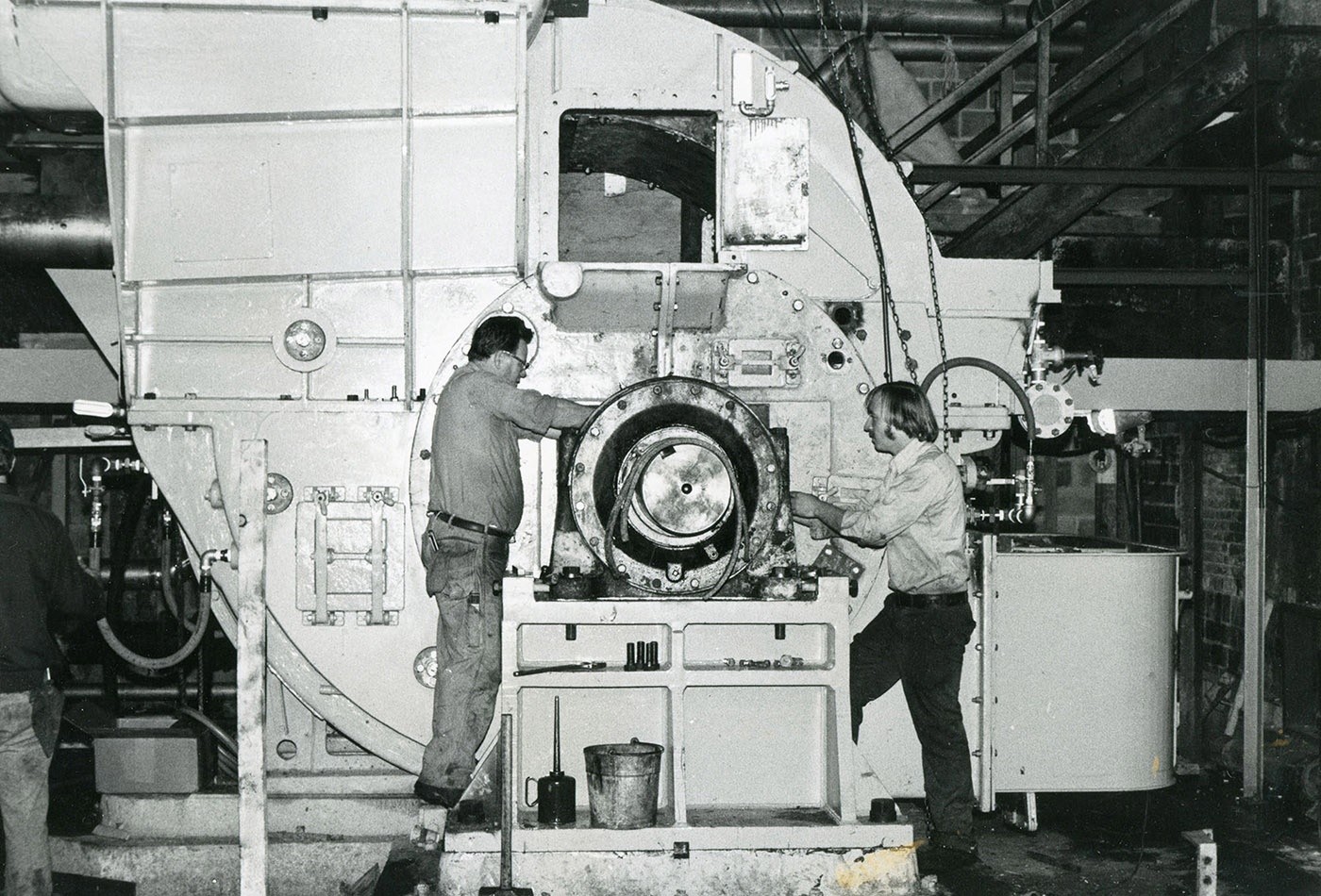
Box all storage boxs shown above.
[64,701,219,795]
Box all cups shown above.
[525,770,575,825]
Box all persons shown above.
[0,417,108,894]
[414,313,597,825]
[788,381,981,860]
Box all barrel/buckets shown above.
[583,737,665,831]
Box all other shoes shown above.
[916,844,978,872]
[413,779,467,809]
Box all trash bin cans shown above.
[584,737,664,830]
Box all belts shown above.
[889,590,968,607]
[430,510,514,540]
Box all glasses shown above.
[501,350,530,370]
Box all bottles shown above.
[550,565,590,601]
[760,567,800,601]
